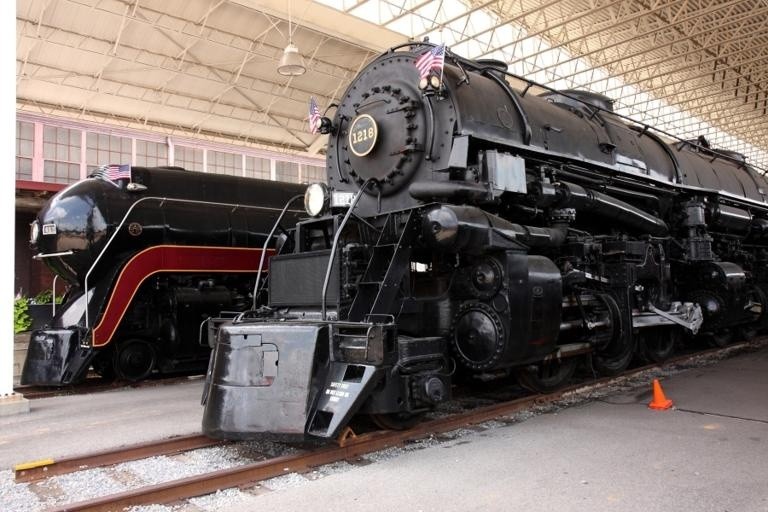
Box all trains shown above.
[199,36,767,447]
[19,163,313,391]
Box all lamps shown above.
[276,0,306,78]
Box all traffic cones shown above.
[649,378,673,409]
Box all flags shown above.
[309,97,322,134]
[103,164,130,180]
[415,45,444,78]
